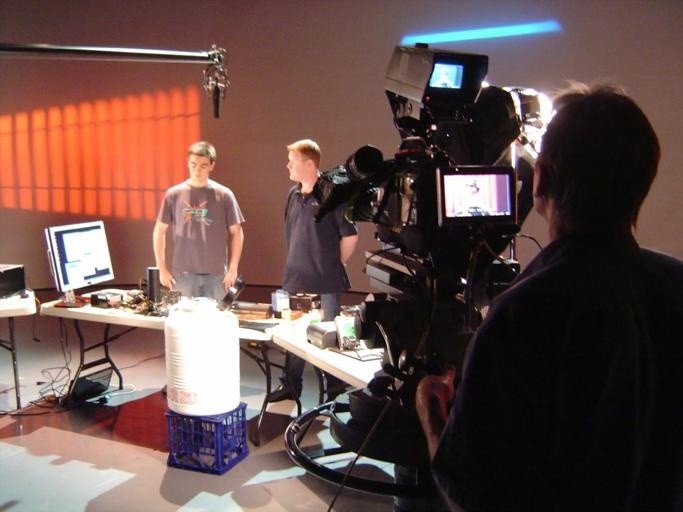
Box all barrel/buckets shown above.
[164,297,241,415]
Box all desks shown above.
[273,310,384,446]
[0,288,37,410]
[40,288,325,446]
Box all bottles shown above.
[297,294,303,310]
[170,291,181,303]
[306,294,319,309]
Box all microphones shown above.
[212,86,221,119]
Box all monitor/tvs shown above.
[44,219,115,308]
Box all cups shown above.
[147,267,161,303]
[300,297,311,312]
[289,296,298,311]
[270,291,290,315]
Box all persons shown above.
[154,142,246,394]
[416,84,683,512]
[270,138,358,407]
